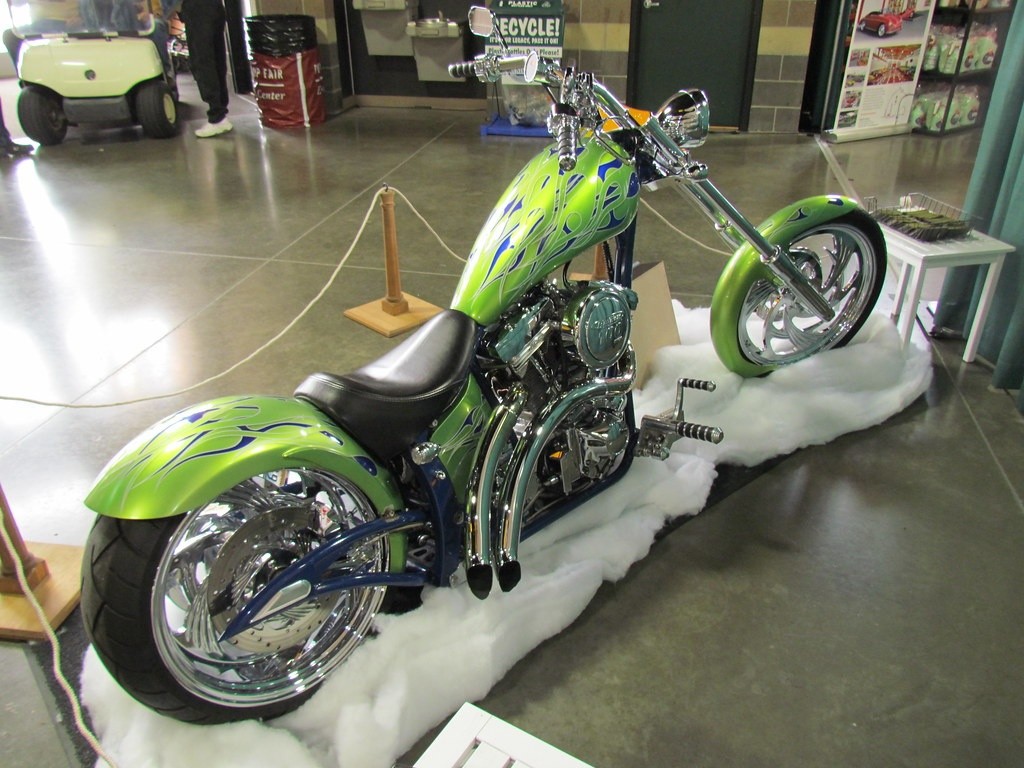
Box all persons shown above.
[0,99,34,156]
[159,0,232,137]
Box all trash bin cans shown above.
[245,15,327,130]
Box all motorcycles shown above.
[80,5,887,723]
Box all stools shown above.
[875,222,1018,361]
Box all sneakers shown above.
[0,142,35,156]
[194,117,233,138]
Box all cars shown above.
[9,0,181,146]
[857,11,904,38]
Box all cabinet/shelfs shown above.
[913,0,1016,138]
